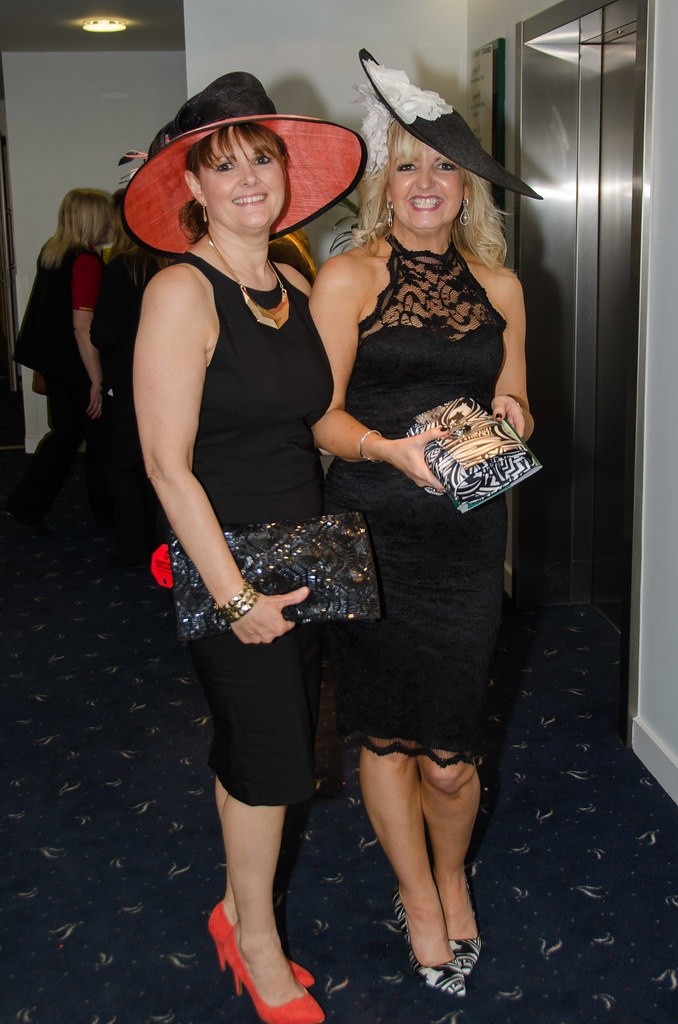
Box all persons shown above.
[126,72,334,1024]
[0,187,117,544]
[92,189,174,558]
[304,97,536,1001]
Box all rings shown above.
[424,482,448,496]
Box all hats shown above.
[358,47,544,201]
[119,71,368,260]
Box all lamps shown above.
[83,18,127,33]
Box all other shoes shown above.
[111,558,151,569]
[0,510,48,533]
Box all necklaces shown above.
[205,231,291,328]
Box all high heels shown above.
[208,901,315,988]
[223,925,325,1024]
[448,873,481,976]
[392,891,466,998]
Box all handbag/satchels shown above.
[168,511,381,639]
[406,396,543,514]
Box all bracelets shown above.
[507,395,521,407]
[212,580,261,623]
[360,429,384,465]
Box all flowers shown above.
[351,58,453,176]
